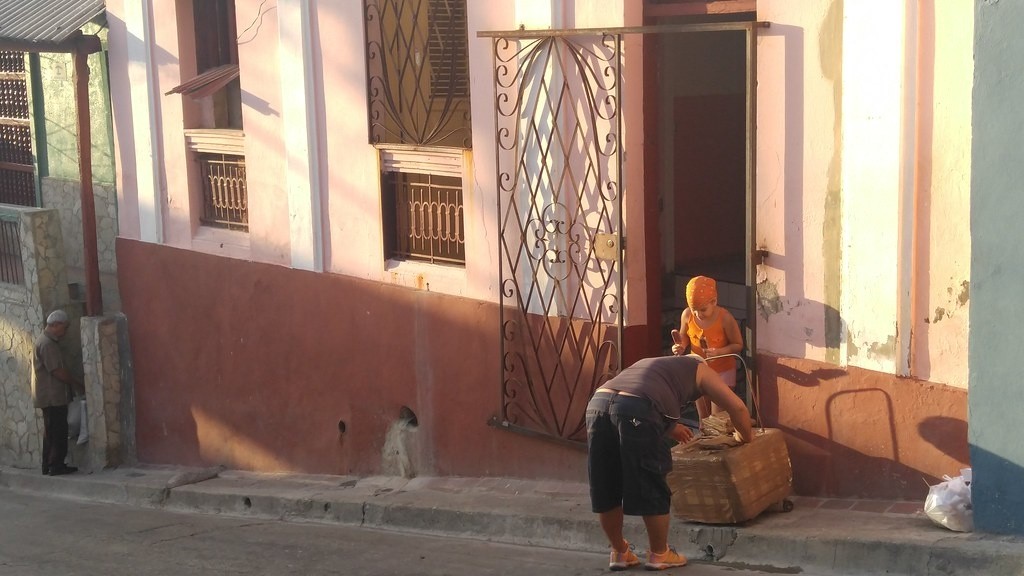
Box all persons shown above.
[585,353,754,572]
[673,274,744,437]
[34,309,80,476]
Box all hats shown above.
[686,276,718,308]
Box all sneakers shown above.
[645,544,687,570]
[610,539,640,570]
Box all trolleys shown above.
[705,355,793,512]
